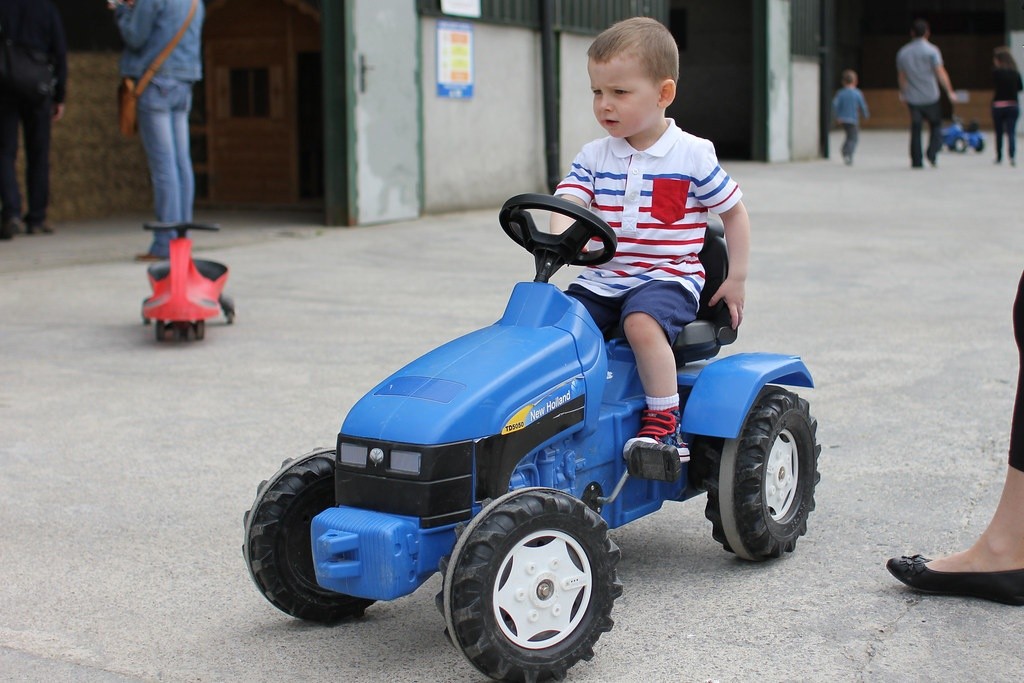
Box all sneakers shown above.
[623,406,690,464]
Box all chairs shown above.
[675,211,737,368]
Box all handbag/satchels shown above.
[0,40,56,102]
[119,77,137,137]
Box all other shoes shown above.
[0,216,17,238]
[135,252,165,262]
[26,220,54,234]
[886,554,1024,606]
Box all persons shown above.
[109,0,206,263]
[832,69,871,166]
[988,46,1023,166]
[549,15,751,461]
[0,0,69,239]
[887,270,1024,609]
[896,20,958,168]
